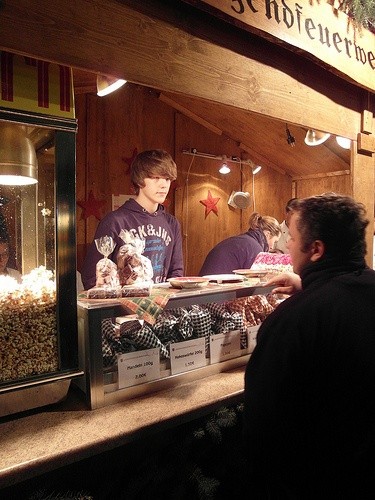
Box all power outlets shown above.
[191,148,197,154]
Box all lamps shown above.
[228,191,251,209]
[97,74,127,97]
[247,158,262,174]
[0,123,38,185]
[219,155,231,175]
[304,129,331,146]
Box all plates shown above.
[233,268,280,277]
[167,276,212,289]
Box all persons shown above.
[244,192,375,500]
[198,211,282,276]
[81,149,184,290]
[0,216,21,278]
[271,198,302,254]
